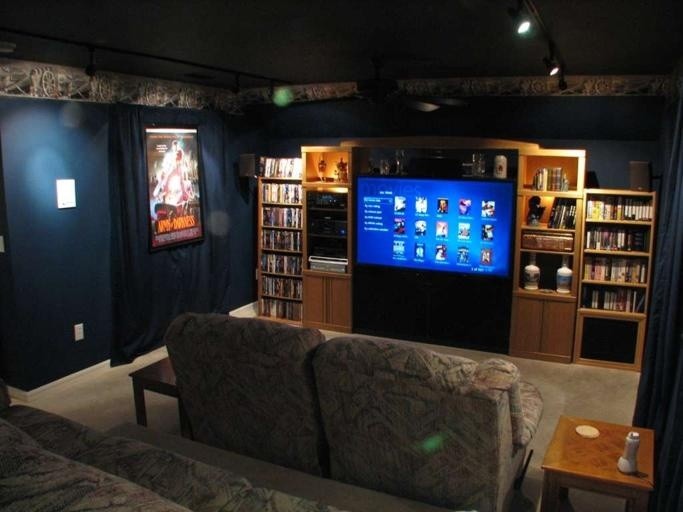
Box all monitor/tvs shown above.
[352,175,517,282]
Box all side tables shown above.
[128,358,180,428]
[541,414,658,511]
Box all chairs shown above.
[311,336,541,508]
[162,314,324,476]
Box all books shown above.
[627,158,653,193]
[257,154,304,323]
[579,197,652,315]
[520,164,577,254]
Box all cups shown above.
[472,152,487,178]
[378,159,391,176]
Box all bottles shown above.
[493,152,508,180]
[617,431,640,473]
[523,252,573,293]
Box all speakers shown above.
[238,153,259,179]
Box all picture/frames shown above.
[141,121,205,253]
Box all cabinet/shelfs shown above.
[509,148,586,362]
[354,141,540,183]
[258,155,302,328]
[301,145,364,334]
[574,189,658,370]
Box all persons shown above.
[151,138,195,215]
